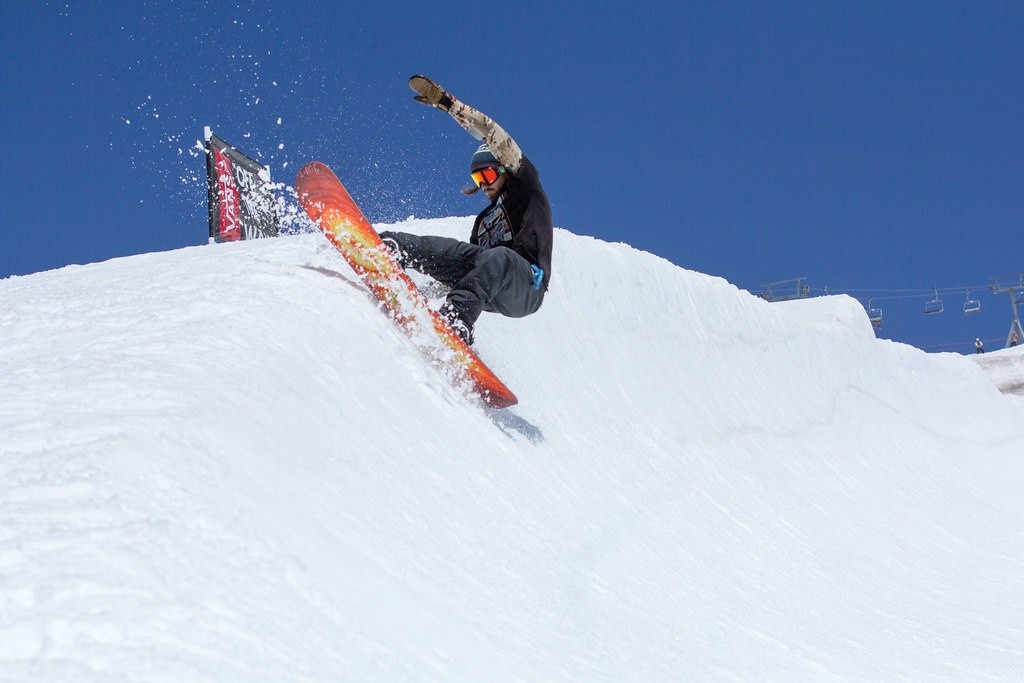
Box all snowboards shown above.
[295,162,519,407]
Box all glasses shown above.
[470,166,504,189]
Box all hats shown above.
[469,144,508,173]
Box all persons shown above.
[377,74,553,346]
[1011,330,1019,347]
[975,338,985,353]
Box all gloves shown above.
[409,75,456,112]
